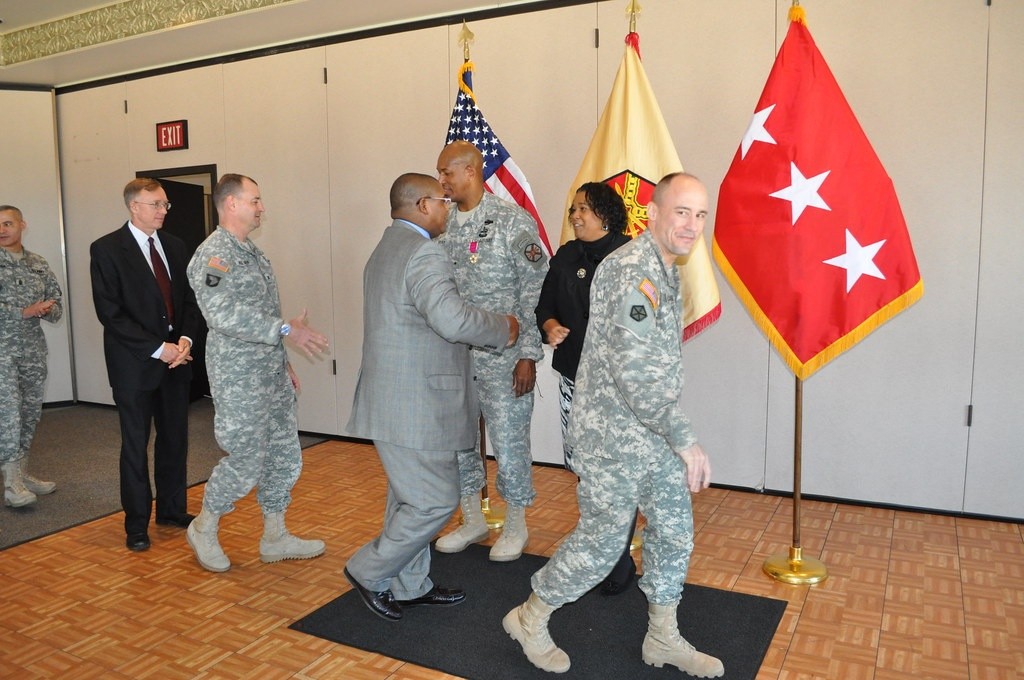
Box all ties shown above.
[148,237,175,323]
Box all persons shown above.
[185,173,330,573]
[504,173,726,679]
[344,174,518,621]
[534,178,636,594]
[430,140,550,560]
[90,178,203,552]
[0,206,63,508]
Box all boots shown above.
[435,494,490,554]
[642,605,725,678]
[261,511,325,563]
[2,463,37,507]
[20,458,57,496]
[186,505,230,572]
[502,592,571,674]
[489,505,529,561]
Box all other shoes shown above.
[600,556,637,595]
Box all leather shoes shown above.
[397,586,467,608]
[343,566,403,622]
[126,532,150,551]
[156,513,196,528]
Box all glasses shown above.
[416,196,452,210]
[135,201,171,210]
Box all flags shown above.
[712,4,924,380]
[444,57,554,262]
[559,33,722,347]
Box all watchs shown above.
[279,320,292,337]
